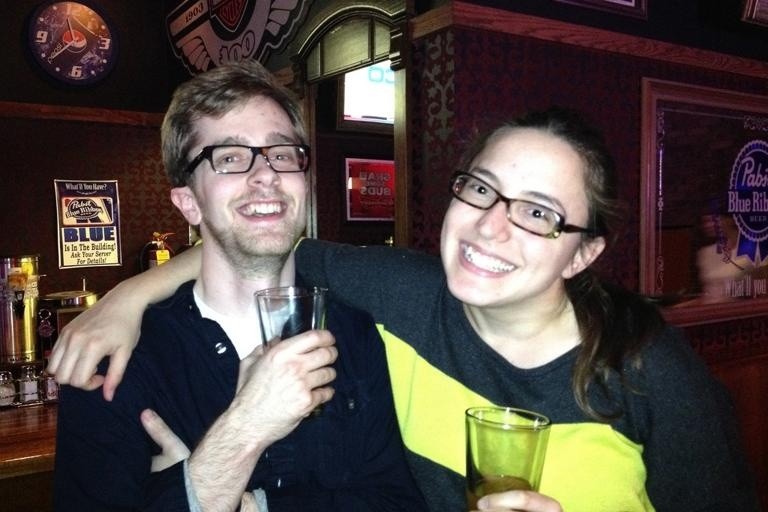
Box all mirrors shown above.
[638,75,768,328]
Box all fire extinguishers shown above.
[141,231,176,269]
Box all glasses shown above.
[179,144,311,185]
[451,173,591,238]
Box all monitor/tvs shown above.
[344,60,395,125]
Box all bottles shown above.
[0,360,59,408]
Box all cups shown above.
[253,284,328,354]
[465,405,552,512]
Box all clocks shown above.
[27,1,120,91]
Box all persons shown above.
[54,56,429,512]
[46,112,762,512]
